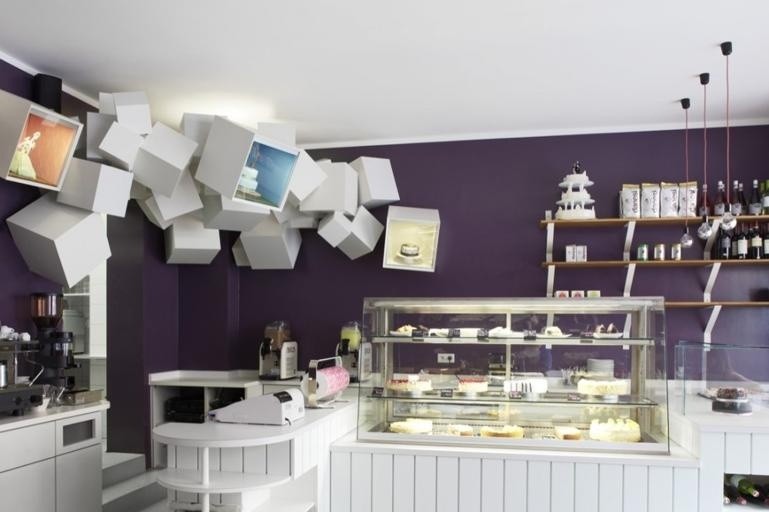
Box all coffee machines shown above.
[30,290,85,393]
[0,340,46,417]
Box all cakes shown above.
[448,424,473,436]
[504,378,548,402]
[480,424,523,438]
[576,378,632,394]
[392,417,433,433]
[387,378,433,393]
[458,376,489,393]
[589,418,641,442]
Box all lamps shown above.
[719,42,739,232]
[699,74,710,238]
[678,96,694,249]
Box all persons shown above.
[9,129,43,180]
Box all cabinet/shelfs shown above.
[1,399,111,512]
[150,423,292,512]
[359,292,671,457]
[148,370,260,473]
[541,210,769,351]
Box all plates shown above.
[536,332,572,339]
[592,332,623,339]
[389,330,411,336]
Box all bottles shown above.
[724,473,769,505]
[711,219,769,259]
[698,180,769,217]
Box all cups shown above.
[0,326,31,341]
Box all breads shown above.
[555,425,581,439]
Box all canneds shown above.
[653,243,665,261]
[637,242,649,260]
[671,242,681,261]
